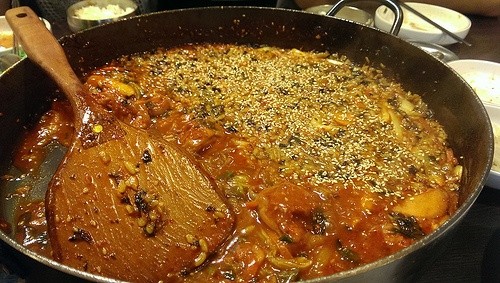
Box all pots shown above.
[0,0,495,283]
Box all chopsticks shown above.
[393,0,471,47]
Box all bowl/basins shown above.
[375,2,471,44]
[304,5,374,28]
[442,59,500,191]
[67,0,138,33]
[0,15,51,58]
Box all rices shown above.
[69,4,135,21]
